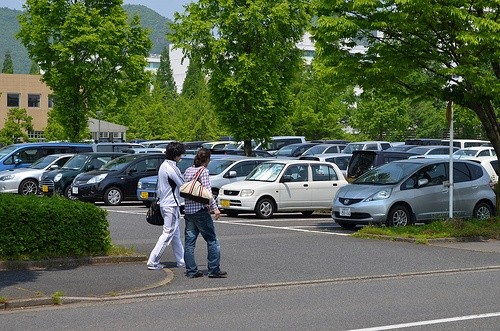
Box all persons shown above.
[147,142,185,269]
[184,148,228,277]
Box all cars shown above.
[69,152,175,206]
[0,143,93,173]
[0,153,110,197]
[332,154,494,233]
[137,155,251,212]
[216,160,350,218]
[38,152,131,200]
[91,135,500,186]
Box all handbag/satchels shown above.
[146,199,164,226]
[179,168,211,205]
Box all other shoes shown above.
[176,261,185,266]
[187,271,204,278]
[208,270,227,277]
[148,264,166,269]
